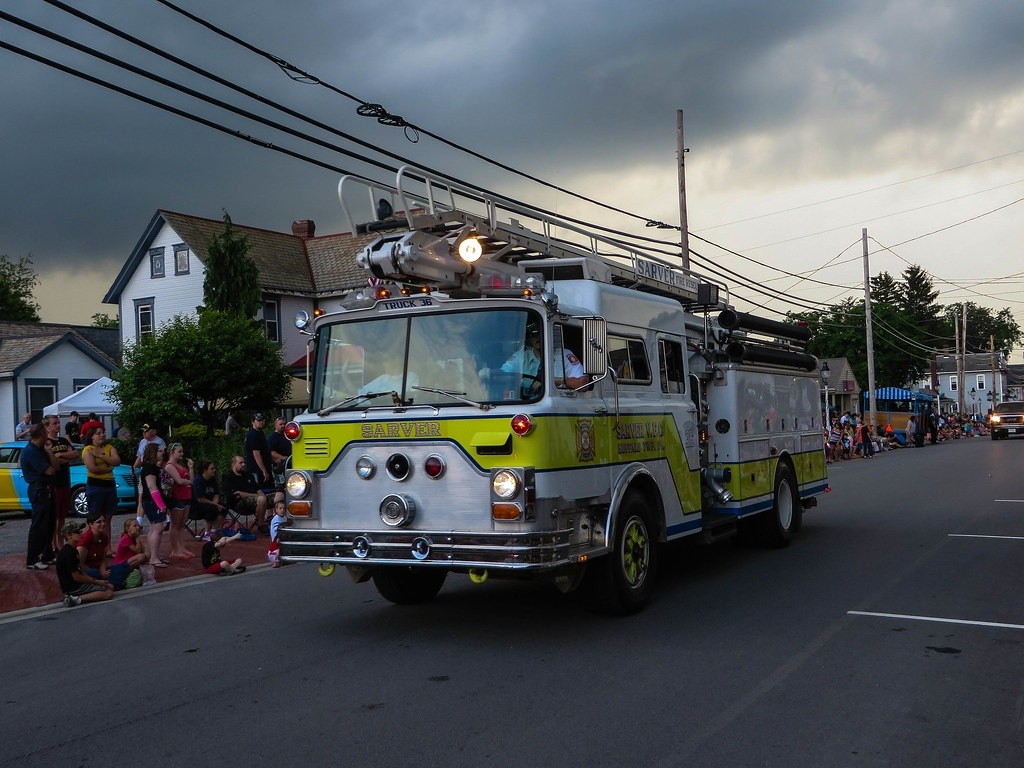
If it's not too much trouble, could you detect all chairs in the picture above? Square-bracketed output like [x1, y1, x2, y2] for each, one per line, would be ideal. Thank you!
[890, 402, 898, 412]
[220, 507, 260, 532]
[183, 512, 208, 542]
[899, 402, 908, 412]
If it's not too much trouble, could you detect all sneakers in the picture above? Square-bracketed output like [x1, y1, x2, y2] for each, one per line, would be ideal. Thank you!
[41, 557, 57, 564]
[27, 561, 48, 570]
[64, 593, 77, 607]
[203, 532, 212, 541]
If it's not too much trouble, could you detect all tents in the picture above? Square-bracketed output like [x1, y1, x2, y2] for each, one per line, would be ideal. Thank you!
[43, 377, 123, 438]
[863, 386, 933, 413]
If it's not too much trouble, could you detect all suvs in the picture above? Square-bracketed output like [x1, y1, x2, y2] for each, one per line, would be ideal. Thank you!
[986, 401, 1024, 439]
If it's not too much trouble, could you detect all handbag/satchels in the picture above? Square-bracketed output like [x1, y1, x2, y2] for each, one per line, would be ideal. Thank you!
[158, 463, 178, 499]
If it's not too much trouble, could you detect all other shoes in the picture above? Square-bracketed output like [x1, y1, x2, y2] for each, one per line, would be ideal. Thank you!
[235, 566, 246, 573]
[264, 515, 274, 525]
[257, 524, 269, 536]
[170, 551, 196, 560]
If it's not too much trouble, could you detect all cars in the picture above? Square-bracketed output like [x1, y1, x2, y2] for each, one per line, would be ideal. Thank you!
[0, 441, 142, 518]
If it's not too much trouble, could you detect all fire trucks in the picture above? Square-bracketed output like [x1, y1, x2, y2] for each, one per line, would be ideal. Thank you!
[272, 164, 842, 617]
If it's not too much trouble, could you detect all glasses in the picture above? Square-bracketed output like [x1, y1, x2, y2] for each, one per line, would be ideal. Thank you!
[70, 530, 80, 534]
[94, 519, 106, 524]
[531, 331, 544, 337]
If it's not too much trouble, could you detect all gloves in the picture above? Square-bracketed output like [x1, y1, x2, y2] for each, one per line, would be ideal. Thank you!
[479, 367, 490, 380]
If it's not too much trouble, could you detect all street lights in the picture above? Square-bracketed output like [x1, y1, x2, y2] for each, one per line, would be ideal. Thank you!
[820, 360, 831, 430]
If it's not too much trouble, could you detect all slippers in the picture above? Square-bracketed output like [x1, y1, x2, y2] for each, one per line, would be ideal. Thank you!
[147, 561, 168, 568]
[148, 558, 170, 565]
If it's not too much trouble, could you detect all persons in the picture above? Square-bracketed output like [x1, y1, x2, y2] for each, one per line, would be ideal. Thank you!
[41, 415, 80, 553]
[822, 410, 905, 462]
[225, 410, 240, 435]
[56, 512, 147, 607]
[270, 499, 287, 540]
[79, 413, 105, 444]
[80, 421, 121, 554]
[479, 324, 588, 399]
[342, 348, 419, 405]
[16, 412, 32, 441]
[187, 461, 227, 538]
[132, 413, 170, 533]
[267, 416, 292, 471]
[201, 530, 246, 576]
[64, 410, 82, 444]
[905, 415, 917, 447]
[243, 413, 276, 522]
[221, 455, 284, 535]
[140, 443, 170, 569]
[20, 423, 59, 570]
[929, 409, 991, 444]
[164, 443, 196, 560]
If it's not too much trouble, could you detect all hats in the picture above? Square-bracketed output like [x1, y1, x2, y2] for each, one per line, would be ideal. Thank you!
[254, 413, 265, 420]
[70, 411, 79, 416]
[61, 521, 86, 535]
[140, 423, 156, 432]
